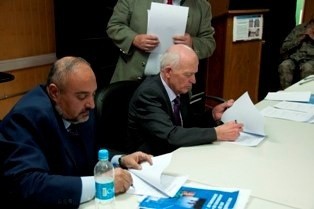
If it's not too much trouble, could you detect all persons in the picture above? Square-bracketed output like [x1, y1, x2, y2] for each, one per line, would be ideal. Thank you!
[106, 0, 216, 84]
[0, 56, 154, 209]
[129, 44, 244, 157]
[278, 19, 314, 90]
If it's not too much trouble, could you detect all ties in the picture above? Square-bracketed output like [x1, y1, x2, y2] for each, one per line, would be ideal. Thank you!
[167, 0, 173, 5]
[173, 96, 181, 126]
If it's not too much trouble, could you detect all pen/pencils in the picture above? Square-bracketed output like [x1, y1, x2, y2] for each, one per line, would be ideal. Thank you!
[113, 162, 135, 188]
[235, 119, 237, 124]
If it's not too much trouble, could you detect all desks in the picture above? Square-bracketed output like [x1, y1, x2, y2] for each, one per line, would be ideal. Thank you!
[79, 74, 314, 209]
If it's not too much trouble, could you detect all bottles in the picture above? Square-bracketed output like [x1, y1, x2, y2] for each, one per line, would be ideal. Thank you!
[95, 149, 116, 209]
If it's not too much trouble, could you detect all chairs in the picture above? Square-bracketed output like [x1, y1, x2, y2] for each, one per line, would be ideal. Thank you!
[179, 58, 226, 128]
[95, 79, 143, 154]
